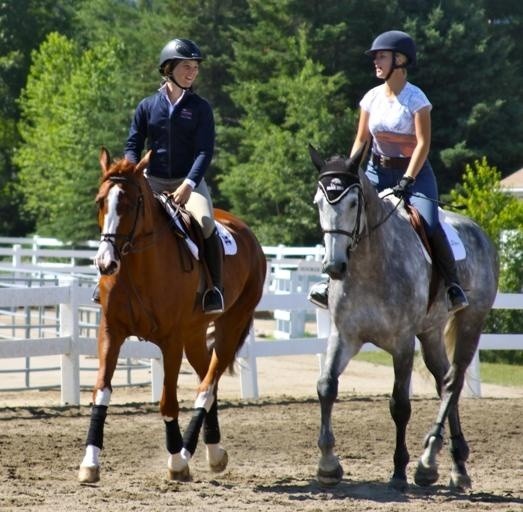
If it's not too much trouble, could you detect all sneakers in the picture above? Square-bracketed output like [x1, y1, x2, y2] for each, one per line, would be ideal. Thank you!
[393, 176, 415, 199]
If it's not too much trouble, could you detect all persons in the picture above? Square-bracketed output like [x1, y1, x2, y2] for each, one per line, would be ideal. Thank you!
[90, 35, 225, 311]
[305, 29, 466, 309]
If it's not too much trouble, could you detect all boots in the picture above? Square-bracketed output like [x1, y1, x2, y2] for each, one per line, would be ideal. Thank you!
[311, 283, 329, 304]
[94, 291, 100, 301]
[427, 221, 465, 306]
[204, 227, 224, 310]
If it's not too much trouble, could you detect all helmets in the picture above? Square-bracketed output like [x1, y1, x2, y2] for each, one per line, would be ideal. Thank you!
[364, 30, 416, 68]
[159, 39, 203, 69]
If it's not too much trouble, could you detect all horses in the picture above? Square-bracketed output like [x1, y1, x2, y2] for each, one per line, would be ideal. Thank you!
[306, 140, 501, 493]
[77, 145, 267, 485]
